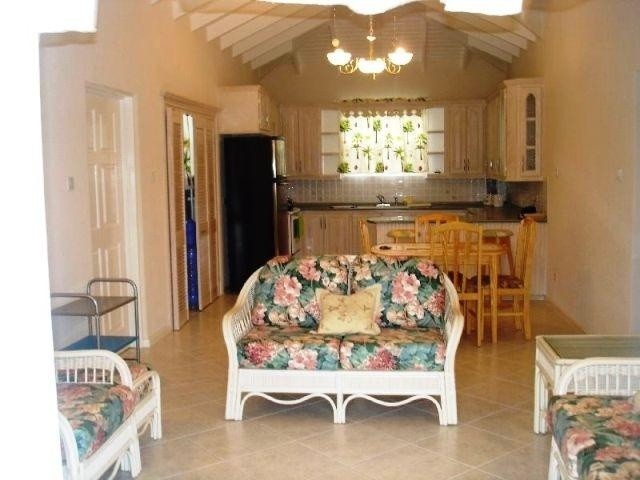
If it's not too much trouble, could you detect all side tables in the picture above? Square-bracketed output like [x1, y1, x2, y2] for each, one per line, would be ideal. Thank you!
[532, 335, 640, 435]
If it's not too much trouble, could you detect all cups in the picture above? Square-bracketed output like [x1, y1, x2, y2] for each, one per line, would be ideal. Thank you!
[494, 194, 503, 207]
[483, 193, 494, 206]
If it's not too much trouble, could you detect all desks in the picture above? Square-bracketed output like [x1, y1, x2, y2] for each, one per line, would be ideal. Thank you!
[371, 240, 505, 345]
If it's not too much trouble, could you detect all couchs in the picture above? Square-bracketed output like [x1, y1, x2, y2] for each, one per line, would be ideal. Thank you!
[221, 253, 464, 427]
[547, 355, 640, 480]
[55, 349, 160, 478]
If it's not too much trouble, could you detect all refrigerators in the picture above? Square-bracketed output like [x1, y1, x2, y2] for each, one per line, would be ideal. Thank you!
[220, 134, 288, 295]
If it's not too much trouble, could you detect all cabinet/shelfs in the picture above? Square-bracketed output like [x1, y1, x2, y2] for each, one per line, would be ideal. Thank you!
[425, 101, 446, 180]
[352, 214, 377, 256]
[51, 277, 141, 366]
[485, 87, 543, 183]
[446, 104, 485, 179]
[303, 213, 350, 257]
[257, 87, 283, 137]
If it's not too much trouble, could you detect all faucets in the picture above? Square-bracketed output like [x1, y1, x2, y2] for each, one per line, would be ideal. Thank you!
[375, 194, 390, 207]
[393, 192, 399, 206]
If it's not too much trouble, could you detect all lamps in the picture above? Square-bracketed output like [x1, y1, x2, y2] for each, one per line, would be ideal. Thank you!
[326, 15, 414, 81]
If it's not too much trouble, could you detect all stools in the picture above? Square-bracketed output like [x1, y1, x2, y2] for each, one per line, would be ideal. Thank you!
[387, 229, 422, 243]
[481, 228, 521, 331]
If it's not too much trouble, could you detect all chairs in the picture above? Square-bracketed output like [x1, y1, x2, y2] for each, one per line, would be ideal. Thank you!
[467, 217, 536, 341]
[415, 213, 459, 243]
[430, 221, 482, 348]
